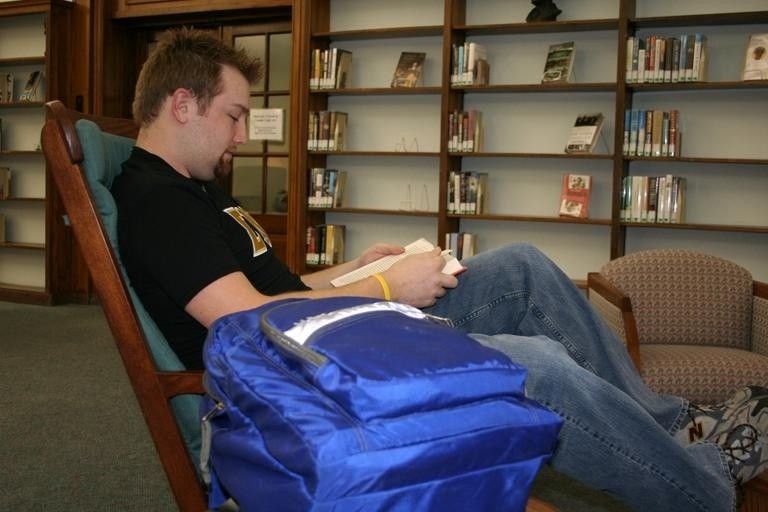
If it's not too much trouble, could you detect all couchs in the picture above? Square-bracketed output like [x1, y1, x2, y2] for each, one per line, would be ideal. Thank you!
[585, 249, 768, 403]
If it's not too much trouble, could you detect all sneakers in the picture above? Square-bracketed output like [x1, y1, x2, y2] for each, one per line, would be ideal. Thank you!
[671, 386, 768, 447]
[708, 396, 768, 485]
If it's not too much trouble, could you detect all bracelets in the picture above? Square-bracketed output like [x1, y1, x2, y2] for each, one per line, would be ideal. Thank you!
[373, 272, 391, 300]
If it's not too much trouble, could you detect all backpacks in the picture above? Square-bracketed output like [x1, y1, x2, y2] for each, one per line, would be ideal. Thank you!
[202, 297, 563, 511]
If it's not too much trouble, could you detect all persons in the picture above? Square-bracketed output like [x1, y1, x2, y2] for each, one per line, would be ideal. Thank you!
[111, 29, 767, 511]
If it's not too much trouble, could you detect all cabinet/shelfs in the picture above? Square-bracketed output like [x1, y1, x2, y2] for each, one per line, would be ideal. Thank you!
[305, 22, 452, 283]
[612, 1, 768, 296]
[434, 0, 635, 286]
[0, 52, 48, 307]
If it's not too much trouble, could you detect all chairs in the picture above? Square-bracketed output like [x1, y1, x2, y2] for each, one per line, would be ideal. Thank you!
[38, 91, 565, 512]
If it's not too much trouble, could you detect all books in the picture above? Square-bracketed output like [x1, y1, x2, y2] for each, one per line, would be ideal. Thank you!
[447, 232, 475, 265]
[447, 170, 487, 214]
[626, 33, 706, 82]
[560, 174, 592, 218]
[541, 41, 576, 82]
[307, 111, 347, 151]
[390, 52, 426, 88]
[310, 48, 351, 89]
[450, 42, 489, 86]
[622, 109, 681, 157]
[328, 238, 467, 288]
[309, 168, 345, 209]
[742, 34, 767, 81]
[619, 174, 686, 222]
[0, 73, 13, 102]
[0, 214, 7, 244]
[448, 108, 482, 152]
[305, 225, 344, 266]
[564, 112, 606, 152]
[0, 167, 11, 197]
[18, 72, 45, 101]
